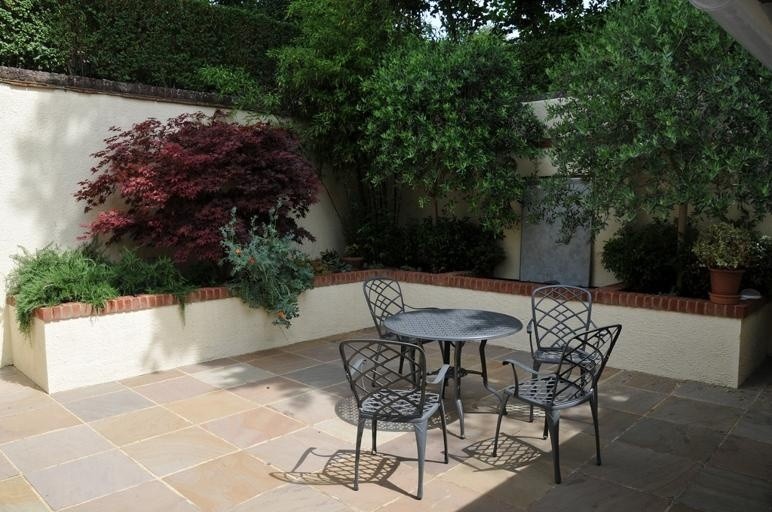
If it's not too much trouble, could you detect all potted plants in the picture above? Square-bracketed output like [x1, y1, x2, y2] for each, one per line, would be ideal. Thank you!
[690, 224, 767, 306]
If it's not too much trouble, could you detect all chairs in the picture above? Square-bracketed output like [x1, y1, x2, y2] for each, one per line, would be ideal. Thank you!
[492, 324, 622, 485]
[362, 276, 441, 398]
[526, 285, 605, 423]
[338, 339, 449, 501]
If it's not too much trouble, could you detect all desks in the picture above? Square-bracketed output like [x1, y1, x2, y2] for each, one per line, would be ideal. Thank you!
[383, 308, 523, 439]
